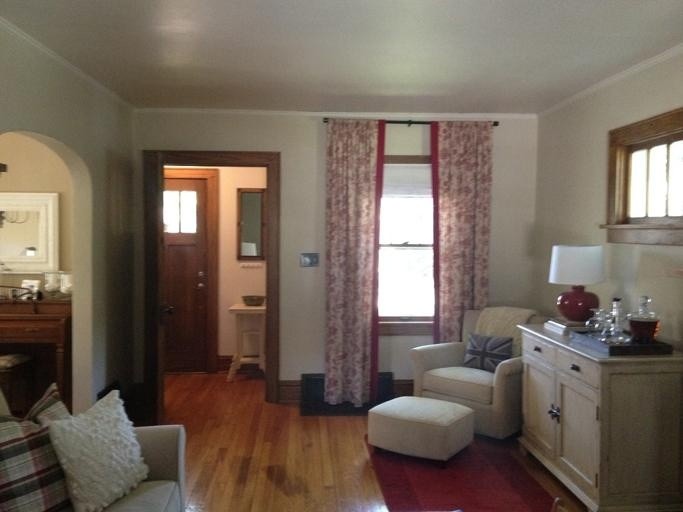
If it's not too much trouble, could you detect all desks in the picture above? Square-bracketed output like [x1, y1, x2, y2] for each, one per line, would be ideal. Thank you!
[0, 291, 72, 409]
[226, 303, 267, 383]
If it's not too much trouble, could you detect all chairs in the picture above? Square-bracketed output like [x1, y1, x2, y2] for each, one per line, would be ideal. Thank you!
[0, 391, 186, 512]
[411, 307, 549, 444]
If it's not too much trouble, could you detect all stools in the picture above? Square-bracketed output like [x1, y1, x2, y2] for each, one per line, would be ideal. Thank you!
[0, 349, 33, 412]
[365, 395, 476, 463]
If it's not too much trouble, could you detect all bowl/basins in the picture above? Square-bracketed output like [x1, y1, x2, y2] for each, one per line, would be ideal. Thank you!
[241, 296, 265, 305]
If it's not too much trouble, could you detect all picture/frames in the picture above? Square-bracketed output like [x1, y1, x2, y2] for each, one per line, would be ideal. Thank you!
[20, 279, 41, 300]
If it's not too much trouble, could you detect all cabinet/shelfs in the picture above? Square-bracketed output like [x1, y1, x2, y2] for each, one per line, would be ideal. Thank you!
[515, 323, 683, 512]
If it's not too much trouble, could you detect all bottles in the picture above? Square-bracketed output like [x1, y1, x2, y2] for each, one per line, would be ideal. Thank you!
[585, 294, 652, 343]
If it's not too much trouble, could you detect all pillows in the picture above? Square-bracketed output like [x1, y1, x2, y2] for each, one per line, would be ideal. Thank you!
[0, 384, 73, 510]
[48, 388, 150, 511]
[465, 332, 514, 373]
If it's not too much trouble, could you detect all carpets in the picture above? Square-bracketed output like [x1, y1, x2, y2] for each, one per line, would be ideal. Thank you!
[362, 430, 562, 512]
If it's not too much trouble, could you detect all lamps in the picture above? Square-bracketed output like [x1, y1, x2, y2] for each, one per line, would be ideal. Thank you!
[0, 211, 35, 228]
[543, 243, 608, 337]
[24, 246, 37, 256]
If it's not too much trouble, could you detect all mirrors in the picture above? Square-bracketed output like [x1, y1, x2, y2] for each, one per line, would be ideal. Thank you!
[0, 190, 60, 274]
[235, 186, 267, 261]
